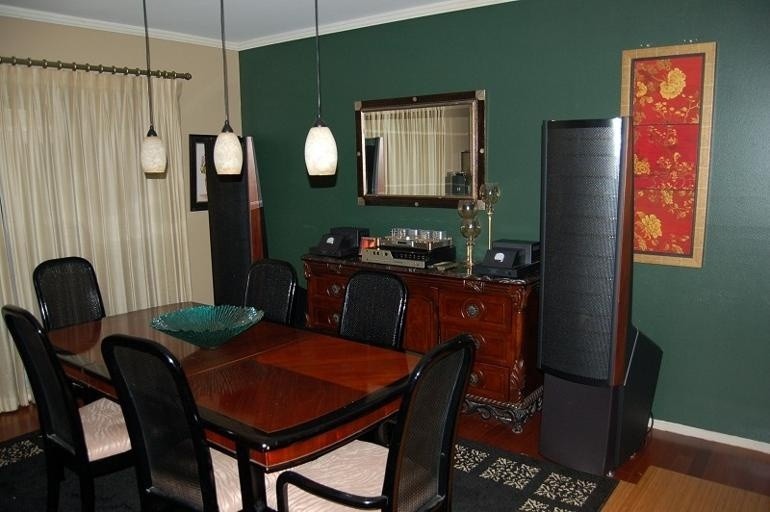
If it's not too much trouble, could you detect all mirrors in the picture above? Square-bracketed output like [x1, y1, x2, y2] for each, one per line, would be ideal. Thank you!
[350, 89, 488, 210]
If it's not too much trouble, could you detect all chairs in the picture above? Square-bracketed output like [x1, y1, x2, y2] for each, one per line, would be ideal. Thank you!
[2, 302, 139, 512]
[259, 332, 477, 512]
[29, 256, 106, 409]
[240, 256, 299, 329]
[338, 266, 409, 353]
[98, 329, 249, 512]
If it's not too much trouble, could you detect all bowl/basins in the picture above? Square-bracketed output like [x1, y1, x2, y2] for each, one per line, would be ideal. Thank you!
[146, 304, 265, 351]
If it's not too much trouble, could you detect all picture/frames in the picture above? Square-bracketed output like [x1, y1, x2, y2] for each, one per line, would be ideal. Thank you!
[186, 132, 211, 213]
[615, 39, 718, 270]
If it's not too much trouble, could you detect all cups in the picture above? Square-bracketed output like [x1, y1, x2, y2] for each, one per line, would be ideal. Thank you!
[377, 227, 448, 249]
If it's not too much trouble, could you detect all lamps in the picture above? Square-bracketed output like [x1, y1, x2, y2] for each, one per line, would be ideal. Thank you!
[137, 0, 170, 177]
[211, 0, 245, 174]
[302, 0, 340, 177]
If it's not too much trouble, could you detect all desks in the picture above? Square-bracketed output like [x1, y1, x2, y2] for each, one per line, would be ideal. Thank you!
[37, 298, 430, 512]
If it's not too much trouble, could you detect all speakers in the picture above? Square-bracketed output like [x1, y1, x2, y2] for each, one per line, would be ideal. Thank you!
[538, 117, 663, 478]
[205, 136, 268, 309]
[364, 137, 385, 195]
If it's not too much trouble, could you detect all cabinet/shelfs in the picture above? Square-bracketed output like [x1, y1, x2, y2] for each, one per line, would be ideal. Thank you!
[298, 245, 546, 438]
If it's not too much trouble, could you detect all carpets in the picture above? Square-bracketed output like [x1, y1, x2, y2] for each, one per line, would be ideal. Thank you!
[0, 415, 622, 512]
